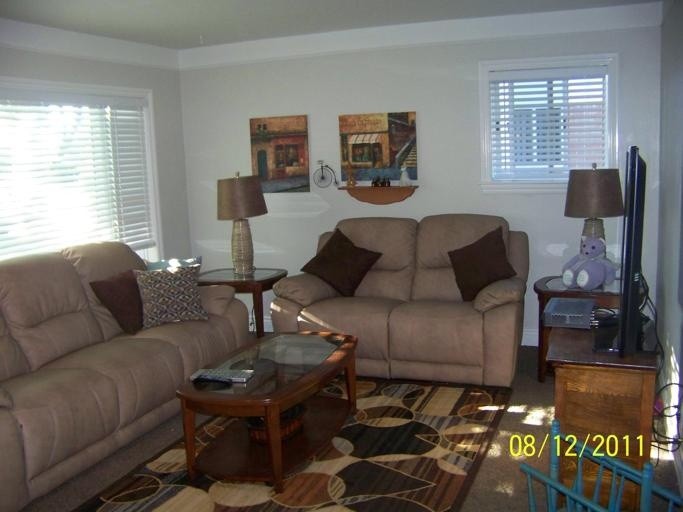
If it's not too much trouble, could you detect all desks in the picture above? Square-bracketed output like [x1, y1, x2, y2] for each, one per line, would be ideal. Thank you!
[197, 269, 288, 338]
[533, 276, 624, 382]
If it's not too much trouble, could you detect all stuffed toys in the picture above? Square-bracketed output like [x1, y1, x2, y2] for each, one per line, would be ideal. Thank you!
[561, 235, 615, 291]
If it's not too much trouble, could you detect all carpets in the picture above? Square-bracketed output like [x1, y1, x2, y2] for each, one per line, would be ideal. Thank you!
[72, 382, 513, 512]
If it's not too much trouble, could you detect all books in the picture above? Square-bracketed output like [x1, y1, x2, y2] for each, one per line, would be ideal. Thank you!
[189, 368, 255, 384]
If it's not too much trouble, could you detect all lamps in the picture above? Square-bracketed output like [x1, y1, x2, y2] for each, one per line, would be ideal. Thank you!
[564, 163, 626, 287]
[217, 171, 268, 275]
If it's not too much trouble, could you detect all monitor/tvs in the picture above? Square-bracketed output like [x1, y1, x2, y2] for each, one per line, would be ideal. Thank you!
[597, 146, 658, 354]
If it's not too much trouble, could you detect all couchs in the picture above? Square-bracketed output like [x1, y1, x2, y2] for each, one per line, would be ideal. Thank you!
[270, 214, 529, 388]
[0, 242, 249, 512]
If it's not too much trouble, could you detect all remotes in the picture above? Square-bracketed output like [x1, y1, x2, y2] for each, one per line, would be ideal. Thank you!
[192, 375, 232, 386]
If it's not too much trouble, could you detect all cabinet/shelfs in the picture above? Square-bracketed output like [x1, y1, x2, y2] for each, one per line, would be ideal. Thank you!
[546, 329, 660, 511]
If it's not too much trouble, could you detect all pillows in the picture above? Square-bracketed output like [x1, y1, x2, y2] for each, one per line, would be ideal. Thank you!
[91, 270, 144, 334]
[146, 256, 202, 269]
[448, 226, 516, 302]
[135, 263, 209, 327]
[300, 229, 381, 297]
